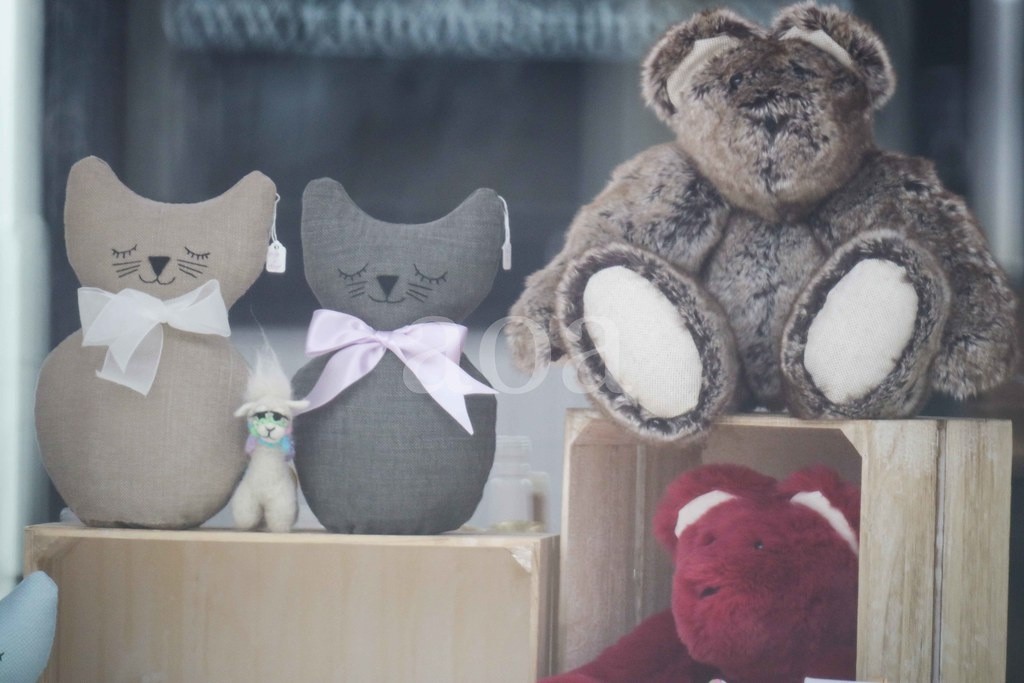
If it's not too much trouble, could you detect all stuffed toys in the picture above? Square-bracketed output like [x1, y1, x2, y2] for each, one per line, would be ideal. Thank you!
[506, 0, 1024, 451]
[231, 357, 309, 532]
[34, 156, 278, 531]
[289, 178, 504, 536]
[542, 464, 861, 683]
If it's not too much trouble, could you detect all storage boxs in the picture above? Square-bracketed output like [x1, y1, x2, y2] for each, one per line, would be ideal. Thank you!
[558, 408, 1013, 683]
